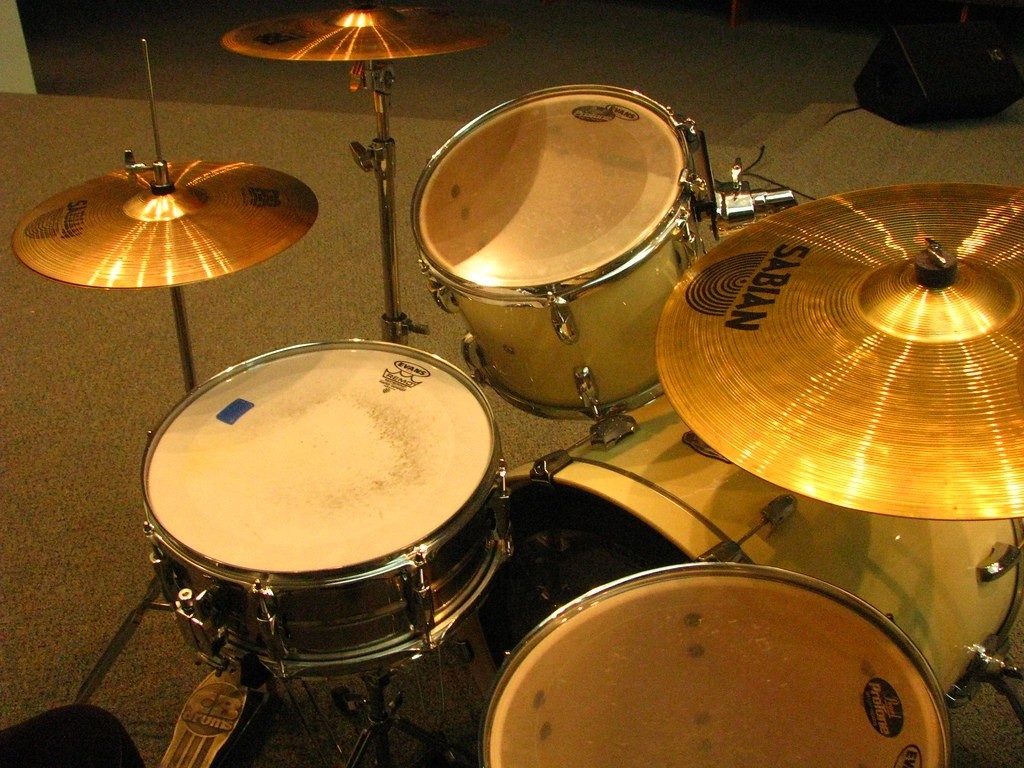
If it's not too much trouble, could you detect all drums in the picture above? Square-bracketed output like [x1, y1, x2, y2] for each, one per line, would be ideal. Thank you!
[410, 82, 712, 422]
[653, 181, 1024, 524]
[476, 391, 1023, 712]
[475, 559, 955, 768]
[137, 338, 513, 679]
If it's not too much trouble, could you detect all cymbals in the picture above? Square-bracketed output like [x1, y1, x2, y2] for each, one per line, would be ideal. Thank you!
[218, 5, 513, 62]
[11, 161, 319, 290]
